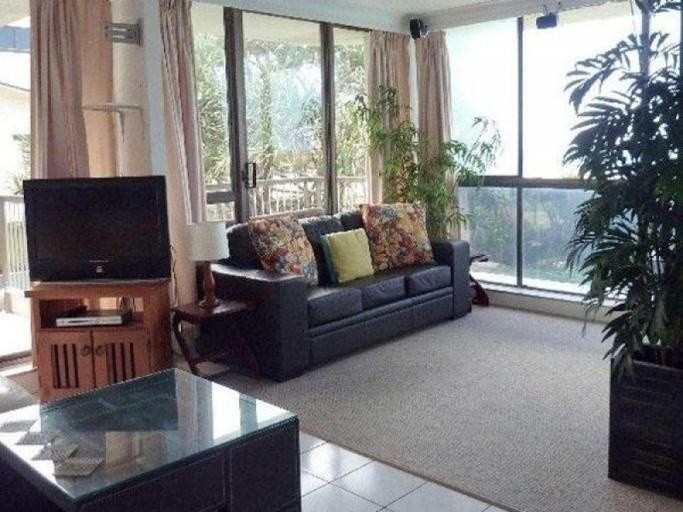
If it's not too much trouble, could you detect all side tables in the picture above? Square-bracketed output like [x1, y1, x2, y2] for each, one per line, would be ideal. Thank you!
[468, 255, 491, 307]
[171, 300, 262, 383]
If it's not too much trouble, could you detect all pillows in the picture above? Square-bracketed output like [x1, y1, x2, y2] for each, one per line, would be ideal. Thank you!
[325, 228, 374, 283]
[318, 236, 336, 283]
[248, 213, 318, 287]
[359, 201, 435, 269]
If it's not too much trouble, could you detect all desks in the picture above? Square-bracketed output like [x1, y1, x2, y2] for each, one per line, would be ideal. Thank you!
[0, 368, 300, 511]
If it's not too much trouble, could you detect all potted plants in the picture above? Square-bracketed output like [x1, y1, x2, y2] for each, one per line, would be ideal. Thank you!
[563, 0, 683, 500]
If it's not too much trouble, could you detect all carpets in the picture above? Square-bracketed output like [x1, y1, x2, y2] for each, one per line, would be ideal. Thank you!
[7, 304, 683, 512]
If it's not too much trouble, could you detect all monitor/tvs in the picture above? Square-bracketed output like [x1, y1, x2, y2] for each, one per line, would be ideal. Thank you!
[21, 173, 173, 289]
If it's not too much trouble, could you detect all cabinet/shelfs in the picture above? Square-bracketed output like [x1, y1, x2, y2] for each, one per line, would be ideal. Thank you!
[24, 278, 171, 402]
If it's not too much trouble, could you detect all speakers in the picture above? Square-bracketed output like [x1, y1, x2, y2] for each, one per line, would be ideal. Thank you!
[409, 17, 422, 40]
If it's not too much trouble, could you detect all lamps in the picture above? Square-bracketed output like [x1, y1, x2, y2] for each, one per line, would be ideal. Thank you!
[535, 2, 561, 28]
[190, 220, 230, 309]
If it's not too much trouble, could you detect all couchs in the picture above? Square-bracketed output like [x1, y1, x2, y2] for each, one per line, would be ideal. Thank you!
[196, 210, 471, 380]
